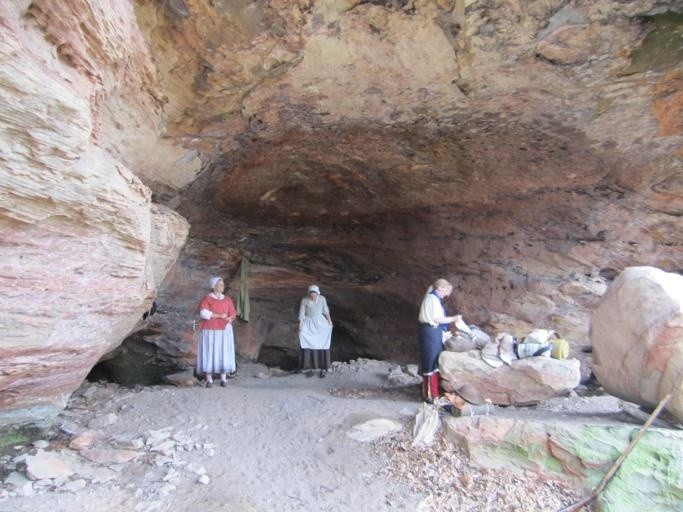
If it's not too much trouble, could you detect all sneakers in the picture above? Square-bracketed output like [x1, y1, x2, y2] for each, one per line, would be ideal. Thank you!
[319, 371, 326, 378]
[220, 381, 227, 386]
[205, 382, 214, 387]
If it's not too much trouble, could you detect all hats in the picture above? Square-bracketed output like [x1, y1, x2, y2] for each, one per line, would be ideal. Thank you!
[307, 284, 321, 295]
[452, 381, 484, 406]
[209, 277, 222, 290]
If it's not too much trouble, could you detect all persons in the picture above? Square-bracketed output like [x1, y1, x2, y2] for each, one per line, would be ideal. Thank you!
[297, 283, 333, 378]
[194, 276, 237, 388]
[417, 277, 462, 405]
